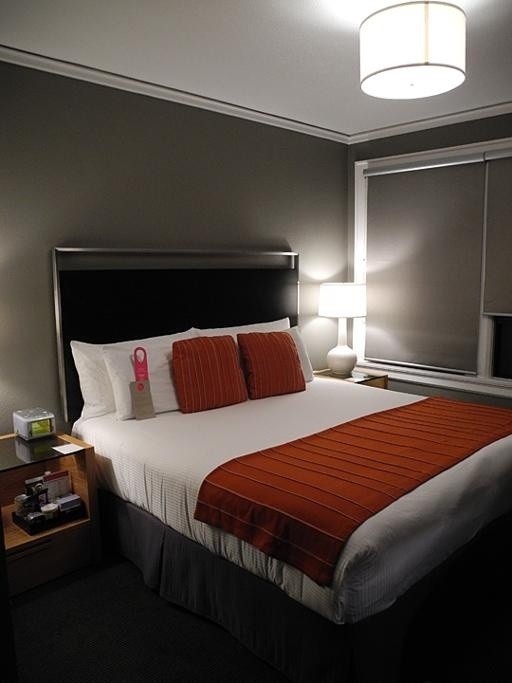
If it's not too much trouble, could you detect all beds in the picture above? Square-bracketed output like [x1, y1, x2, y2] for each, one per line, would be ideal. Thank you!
[54, 247, 512, 683]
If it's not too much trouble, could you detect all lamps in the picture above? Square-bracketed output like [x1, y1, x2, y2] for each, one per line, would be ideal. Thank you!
[359, 0, 468, 101]
[318, 282, 367, 374]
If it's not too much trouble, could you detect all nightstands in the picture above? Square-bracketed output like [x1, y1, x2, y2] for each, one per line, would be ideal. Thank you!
[317, 367, 389, 389]
[0, 428, 99, 597]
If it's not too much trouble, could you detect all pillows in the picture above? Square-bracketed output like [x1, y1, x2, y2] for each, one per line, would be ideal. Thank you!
[69, 317, 313, 417]
